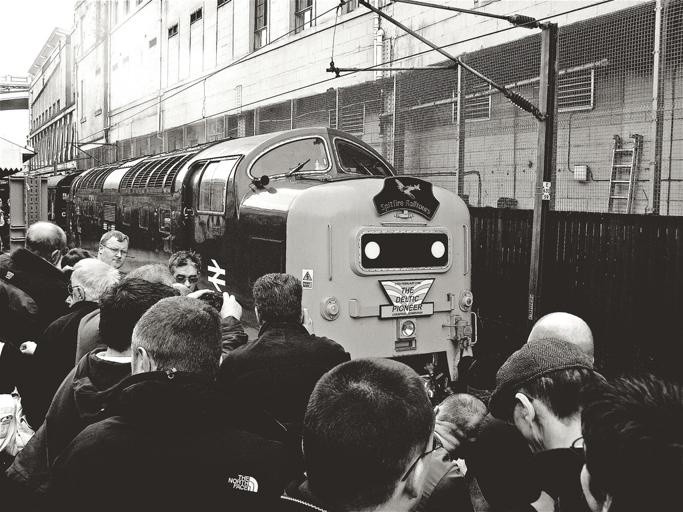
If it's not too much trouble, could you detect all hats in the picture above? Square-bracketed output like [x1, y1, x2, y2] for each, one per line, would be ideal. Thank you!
[483, 339, 596, 418]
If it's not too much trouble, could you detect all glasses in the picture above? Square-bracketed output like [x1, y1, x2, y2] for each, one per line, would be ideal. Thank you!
[103, 244, 128, 255]
[66, 284, 81, 294]
[176, 274, 201, 283]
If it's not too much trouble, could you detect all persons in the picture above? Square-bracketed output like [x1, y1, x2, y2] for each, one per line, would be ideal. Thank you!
[1, 192, 680, 510]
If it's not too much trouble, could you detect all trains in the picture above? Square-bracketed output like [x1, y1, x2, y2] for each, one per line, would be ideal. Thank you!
[46, 125, 479, 401]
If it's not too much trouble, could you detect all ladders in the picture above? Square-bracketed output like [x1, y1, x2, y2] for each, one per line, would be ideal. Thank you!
[608, 134, 643, 214]
